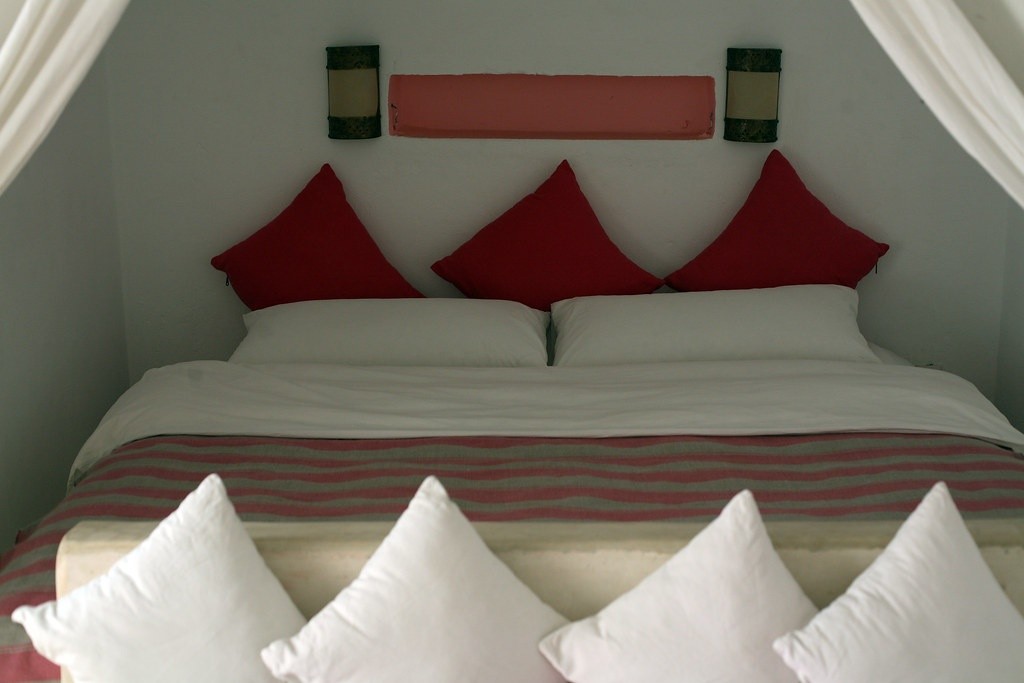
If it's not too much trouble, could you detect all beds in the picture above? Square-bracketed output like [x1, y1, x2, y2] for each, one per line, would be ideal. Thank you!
[0, 138, 1024, 683]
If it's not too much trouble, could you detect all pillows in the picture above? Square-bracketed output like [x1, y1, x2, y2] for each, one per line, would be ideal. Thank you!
[10, 473, 308, 683]
[227, 298, 552, 368]
[209, 163, 426, 311]
[772, 481, 1024, 683]
[553, 284, 880, 372]
[430, 159, 662, 313]
[260, 474, 573, 683]
[538, 489, 820, 683]
[663, 148, 889, 291]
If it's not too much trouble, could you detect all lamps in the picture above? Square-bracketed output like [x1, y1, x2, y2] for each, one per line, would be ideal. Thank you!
[723, 48, 783, 142]
[325, 46, 384, 140]
